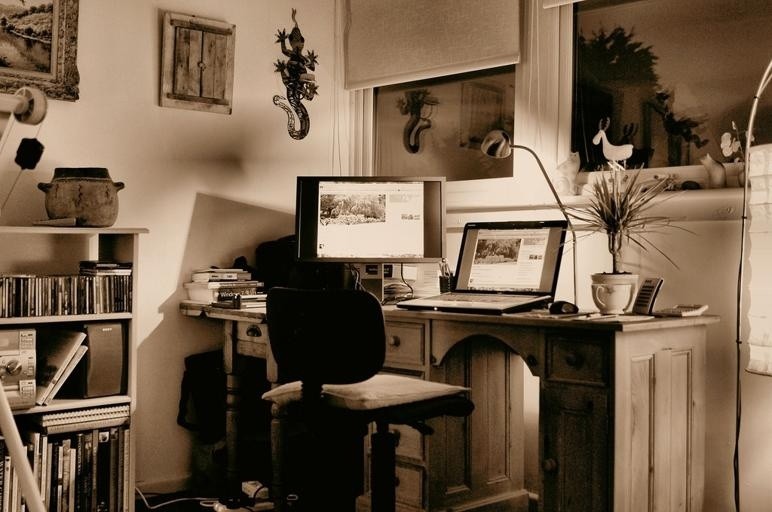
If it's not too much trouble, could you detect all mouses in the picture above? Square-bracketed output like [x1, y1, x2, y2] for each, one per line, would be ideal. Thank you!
[549, 301, 578, 316]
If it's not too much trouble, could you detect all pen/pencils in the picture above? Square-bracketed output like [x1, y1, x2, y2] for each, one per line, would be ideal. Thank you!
[438, 258, 454, 277]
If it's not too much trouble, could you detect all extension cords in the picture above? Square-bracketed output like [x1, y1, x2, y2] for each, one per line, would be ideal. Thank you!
[212, 494, 274, 511]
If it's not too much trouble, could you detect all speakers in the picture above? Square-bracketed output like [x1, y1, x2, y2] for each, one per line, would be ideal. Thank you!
[70, 323, 123, 397]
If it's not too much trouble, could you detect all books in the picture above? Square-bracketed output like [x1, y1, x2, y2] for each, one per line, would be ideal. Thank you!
[184, 267, 269, 310]
[0, 405, 129, 512]
[35, 332, 89, 405]
[0, 261, 132, 319]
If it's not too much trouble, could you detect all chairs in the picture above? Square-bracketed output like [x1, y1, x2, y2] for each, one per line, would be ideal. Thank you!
[261, 287, 475, 512]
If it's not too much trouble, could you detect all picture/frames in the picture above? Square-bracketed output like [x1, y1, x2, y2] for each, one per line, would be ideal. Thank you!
[1, 0, 80, 101]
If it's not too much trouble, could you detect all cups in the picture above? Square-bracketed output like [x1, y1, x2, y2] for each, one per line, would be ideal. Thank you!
[592, 284, 633, 316]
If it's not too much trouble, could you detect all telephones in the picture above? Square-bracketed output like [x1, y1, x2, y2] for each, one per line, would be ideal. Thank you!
[384, 282, 412, 292]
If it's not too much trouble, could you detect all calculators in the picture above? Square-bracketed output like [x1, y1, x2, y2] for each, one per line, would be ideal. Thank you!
[653, 304, 709, 316]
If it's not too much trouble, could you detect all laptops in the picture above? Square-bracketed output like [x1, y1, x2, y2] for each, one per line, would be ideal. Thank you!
[395, 220, 569, 315]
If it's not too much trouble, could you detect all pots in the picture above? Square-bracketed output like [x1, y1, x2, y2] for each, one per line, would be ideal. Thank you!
[38, 168, 124, 227]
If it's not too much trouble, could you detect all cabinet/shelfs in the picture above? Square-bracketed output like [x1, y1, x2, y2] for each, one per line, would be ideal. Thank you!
[0, 226, 149, 512]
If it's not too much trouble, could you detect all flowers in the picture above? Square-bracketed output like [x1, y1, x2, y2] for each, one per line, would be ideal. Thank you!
[558, 164, 702, 274]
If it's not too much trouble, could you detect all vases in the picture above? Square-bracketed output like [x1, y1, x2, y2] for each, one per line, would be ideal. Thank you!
[590, 274, 639, 314]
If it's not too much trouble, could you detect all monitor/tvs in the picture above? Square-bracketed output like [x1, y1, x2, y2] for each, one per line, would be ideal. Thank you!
[294, 176, 448, 302]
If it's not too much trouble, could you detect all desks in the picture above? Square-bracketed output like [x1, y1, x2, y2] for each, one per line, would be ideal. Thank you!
[178, 301, 722, 511]
